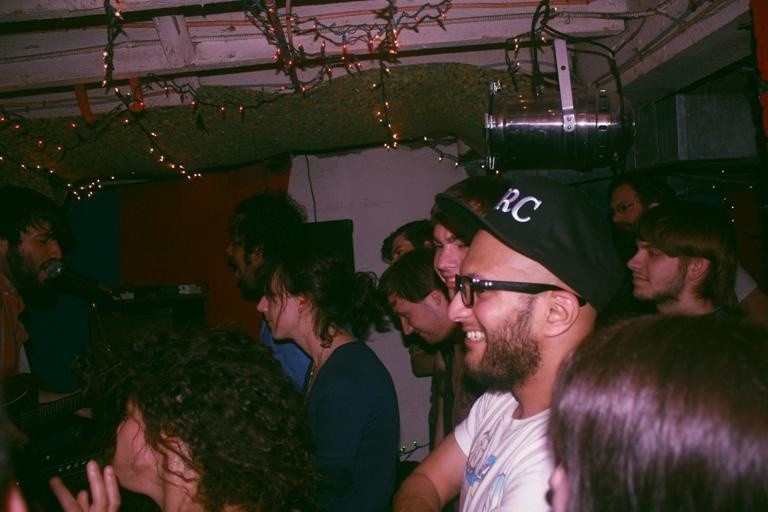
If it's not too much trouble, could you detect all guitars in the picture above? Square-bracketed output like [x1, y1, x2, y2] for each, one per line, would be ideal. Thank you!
[0, 369, 109, 478]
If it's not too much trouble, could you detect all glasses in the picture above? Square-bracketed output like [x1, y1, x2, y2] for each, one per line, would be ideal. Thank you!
[455, 274, 587, 308]
[616, 201, 642, 212]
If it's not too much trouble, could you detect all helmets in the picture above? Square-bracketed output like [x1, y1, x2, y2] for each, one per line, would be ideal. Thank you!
[435, 177, 506, 218]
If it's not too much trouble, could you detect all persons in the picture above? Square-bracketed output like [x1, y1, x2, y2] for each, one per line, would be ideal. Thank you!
[0, 185, 401, 511]
[375, 169, 767, 512]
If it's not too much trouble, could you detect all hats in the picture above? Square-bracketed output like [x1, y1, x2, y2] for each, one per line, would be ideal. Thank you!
[474, 177, 627, 312]
